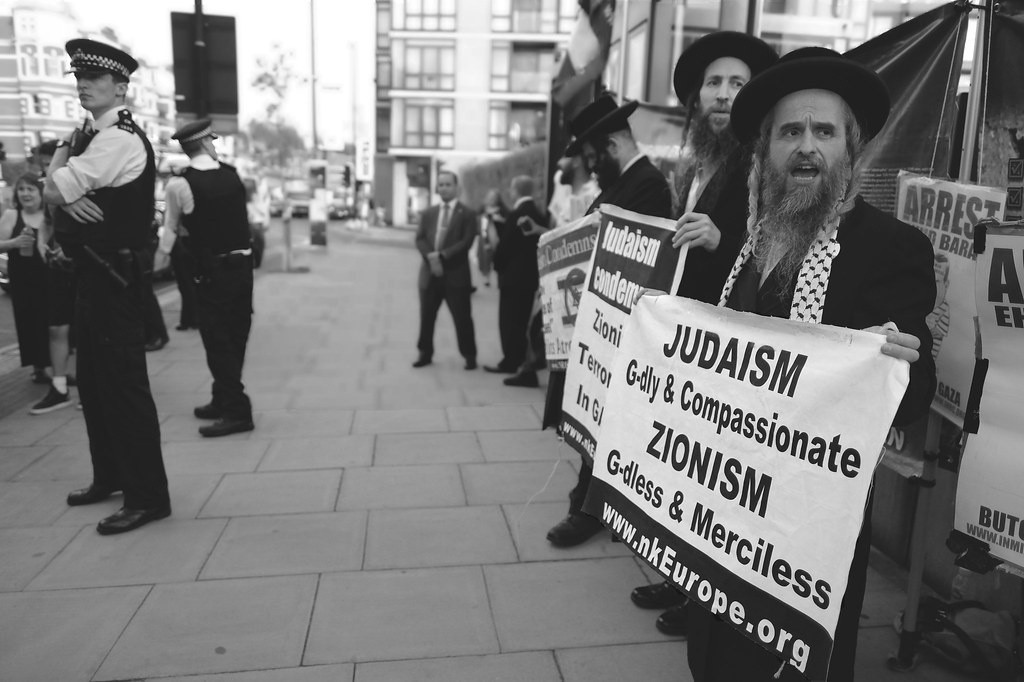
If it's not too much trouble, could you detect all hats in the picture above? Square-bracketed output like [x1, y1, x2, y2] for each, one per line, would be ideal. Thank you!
[727, 48, 891, 146]
[559, 95, 642, 158]
[171, 118, 219, 143]
[673, 30, 781, 113]
[64, 38, 139, 83]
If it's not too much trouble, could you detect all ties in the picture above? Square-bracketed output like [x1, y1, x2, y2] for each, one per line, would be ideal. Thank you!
[436, 206, 450, 251]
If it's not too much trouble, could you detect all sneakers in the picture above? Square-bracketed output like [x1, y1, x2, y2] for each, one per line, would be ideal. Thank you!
[29, 383, 73, 415]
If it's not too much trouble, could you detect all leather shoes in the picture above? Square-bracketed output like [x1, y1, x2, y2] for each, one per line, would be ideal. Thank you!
[195, 399, 227, 418]
[66, 479, 124, 506]
[655, 608, 690, 637]
[199, 414, 255, 437]
[96, 504, 172, 535]
[547, 512, 603, 546]
[630, 580, 687, 611]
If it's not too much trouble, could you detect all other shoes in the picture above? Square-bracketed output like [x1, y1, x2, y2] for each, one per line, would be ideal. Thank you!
[465, 358, 477, 370]
[412, 355, 432, 366]
[484, 363, 517, 374]
[503, 373, 539, 387]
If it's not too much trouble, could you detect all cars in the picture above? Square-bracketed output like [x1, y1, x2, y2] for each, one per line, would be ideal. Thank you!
[0, 147, 358, 297]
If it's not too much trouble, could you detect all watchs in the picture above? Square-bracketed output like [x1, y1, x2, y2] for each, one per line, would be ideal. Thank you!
[56, 140, 70, 148]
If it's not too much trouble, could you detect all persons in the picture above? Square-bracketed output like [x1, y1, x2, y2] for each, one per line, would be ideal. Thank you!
[630, 31, 778, 637]
[485, 175, 543, 388]
[546, 97, 673, 548]
[162, 119, 256, 437]
[475, 187, 506, 286]
[0, 140, 83, 413]
[411, 171, 478, 370]
[504, 142, 602, 388]
[43, 38, 172, 535]
[632, 46, 937, 682]
